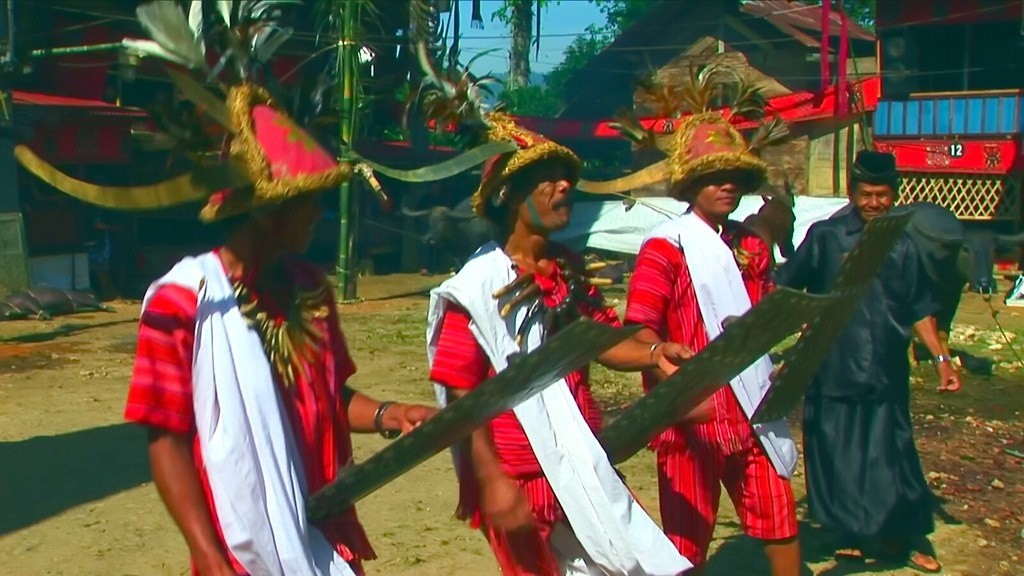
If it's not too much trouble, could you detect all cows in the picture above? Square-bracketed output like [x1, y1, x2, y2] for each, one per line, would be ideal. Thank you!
[897, 202, 1024, 369]
[0, 290, 117, 320]
[402, 195, 499, 274]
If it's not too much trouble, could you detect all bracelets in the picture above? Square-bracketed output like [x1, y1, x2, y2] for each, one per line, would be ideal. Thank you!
[651, 341, 664, 368]
[933, 354, 950, 365]
[373, 401, 403, 439]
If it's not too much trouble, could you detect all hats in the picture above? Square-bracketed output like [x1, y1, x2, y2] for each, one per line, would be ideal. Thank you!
[577, 42, 793, 204]
[851, 150, 897, 185]
[348, 41, 582, 223]
[11, 0, 357, 226]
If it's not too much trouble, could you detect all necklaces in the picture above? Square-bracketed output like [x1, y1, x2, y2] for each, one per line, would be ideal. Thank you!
[491, 251, 624, 351]
[222, 246, 332, 388]
[726, 231, 764, 272]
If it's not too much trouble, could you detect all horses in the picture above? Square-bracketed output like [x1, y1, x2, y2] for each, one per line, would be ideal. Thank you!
[742, 194, 796, 272]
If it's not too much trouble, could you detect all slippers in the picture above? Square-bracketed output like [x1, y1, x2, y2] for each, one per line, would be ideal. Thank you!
[833, 545, 865, 566]
[903, 549, 942, 573]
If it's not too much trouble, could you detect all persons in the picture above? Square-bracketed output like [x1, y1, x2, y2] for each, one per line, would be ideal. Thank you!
[130, 119, 449, 576]
[426, 136, 697, 576]
[623, 121, 813, 576]
[769, 149, 964, 572]
